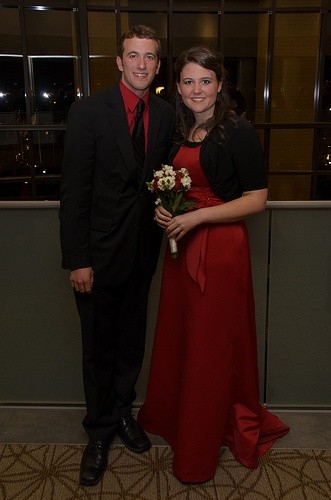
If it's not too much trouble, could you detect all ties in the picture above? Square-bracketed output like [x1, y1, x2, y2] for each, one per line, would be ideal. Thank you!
[131, 99, 146, 183]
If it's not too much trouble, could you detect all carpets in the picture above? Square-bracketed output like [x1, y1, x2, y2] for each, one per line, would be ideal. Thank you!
[0, 443, 331, 500]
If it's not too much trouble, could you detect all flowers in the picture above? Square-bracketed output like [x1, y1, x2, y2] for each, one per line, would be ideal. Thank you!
[146, 165, 196, 259]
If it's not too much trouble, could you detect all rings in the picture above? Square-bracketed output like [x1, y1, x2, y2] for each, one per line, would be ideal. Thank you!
[153, 214, 158, 221]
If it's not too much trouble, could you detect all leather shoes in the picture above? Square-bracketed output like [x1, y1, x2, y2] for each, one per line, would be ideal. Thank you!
[117, 416, 152, 452]
[79, 438, 110, 487]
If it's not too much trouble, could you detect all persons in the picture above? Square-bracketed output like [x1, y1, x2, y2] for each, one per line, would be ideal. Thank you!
[58, 24, 176, 487]
[153, 45, 291, 485]
